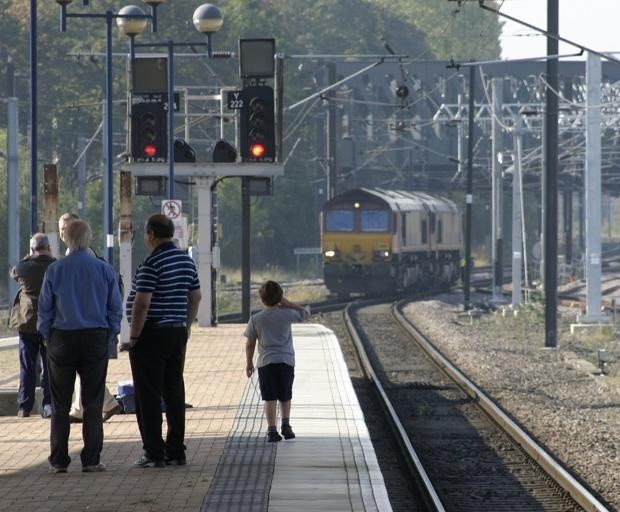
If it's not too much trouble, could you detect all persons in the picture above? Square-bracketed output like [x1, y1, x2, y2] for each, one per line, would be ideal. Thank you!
[119, 214, 201, 467]
[244, 281, 311, 442]
[10, 233, 57, 418]
[36, 220, 123, 472]
[59, 213, 123, 423]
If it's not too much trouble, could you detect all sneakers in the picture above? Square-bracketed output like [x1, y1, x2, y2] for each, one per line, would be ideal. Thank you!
[48, 464, 67, 472]
[267, 431, 282, 442]
[82, 462, 106, 473]
[134, 447, 187, 467]
[17, 407, 30, 417]
[281, 425, 296, 440]
[40, 404, 51, 418]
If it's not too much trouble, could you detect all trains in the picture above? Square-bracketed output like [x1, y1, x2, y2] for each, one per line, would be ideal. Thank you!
[320, 186, 465, 302]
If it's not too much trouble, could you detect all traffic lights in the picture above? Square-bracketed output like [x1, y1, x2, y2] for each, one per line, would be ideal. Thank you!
[237, 84, 274, 159]
[129, 99, 168, 160]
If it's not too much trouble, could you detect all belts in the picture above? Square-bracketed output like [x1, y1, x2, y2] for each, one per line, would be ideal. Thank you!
[148, 322, 188, 330]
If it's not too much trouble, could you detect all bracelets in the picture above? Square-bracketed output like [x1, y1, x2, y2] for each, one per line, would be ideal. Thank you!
[130, 337, 138, 340]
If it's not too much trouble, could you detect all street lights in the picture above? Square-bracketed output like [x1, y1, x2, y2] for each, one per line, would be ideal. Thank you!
[116, 3, 225, 203]
